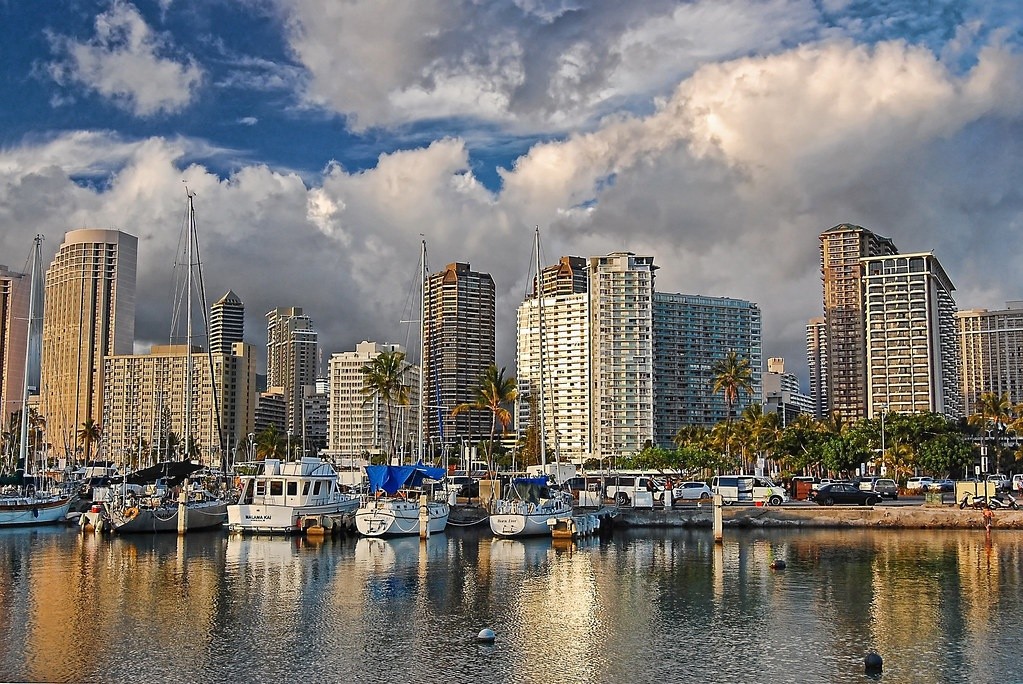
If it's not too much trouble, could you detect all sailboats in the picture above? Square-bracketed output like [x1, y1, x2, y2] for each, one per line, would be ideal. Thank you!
[355, 233, 450, 537]
[488, 225, 574, 536]
[0, 181, 235, 536]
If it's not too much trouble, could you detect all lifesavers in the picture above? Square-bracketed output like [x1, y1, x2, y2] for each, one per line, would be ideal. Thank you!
[125, 507, 138, 519]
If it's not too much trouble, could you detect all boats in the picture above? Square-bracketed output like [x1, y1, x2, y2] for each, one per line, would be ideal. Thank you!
[222, 456, 359, 533]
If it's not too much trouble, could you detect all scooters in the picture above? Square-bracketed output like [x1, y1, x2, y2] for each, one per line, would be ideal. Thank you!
[959, 490, 986, 509]
[988, 491, 1020, 511]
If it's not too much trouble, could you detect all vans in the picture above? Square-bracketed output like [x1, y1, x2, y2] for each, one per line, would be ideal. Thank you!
[711, 475, 789, 506]
[859, 477, 881, 492]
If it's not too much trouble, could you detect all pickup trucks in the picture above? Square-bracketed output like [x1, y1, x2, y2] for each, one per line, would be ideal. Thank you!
[604, 476, 683, 506]
[987, 474, 1023, 491]
[792, 476, 860, 490]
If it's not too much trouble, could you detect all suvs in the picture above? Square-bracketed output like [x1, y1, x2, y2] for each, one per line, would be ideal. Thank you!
[873, 479, 898, 500]
[907, 477, 954, 491]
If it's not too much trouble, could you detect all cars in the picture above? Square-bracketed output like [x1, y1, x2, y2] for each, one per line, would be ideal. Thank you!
[810, 483, 883, 506]
[675, 481, 712, 500]
[440, 476, 474, 492]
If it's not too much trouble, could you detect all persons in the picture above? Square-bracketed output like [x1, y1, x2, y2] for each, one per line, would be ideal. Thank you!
[647, 475, 657, 501]
[1017, 479, 1023, 498]
